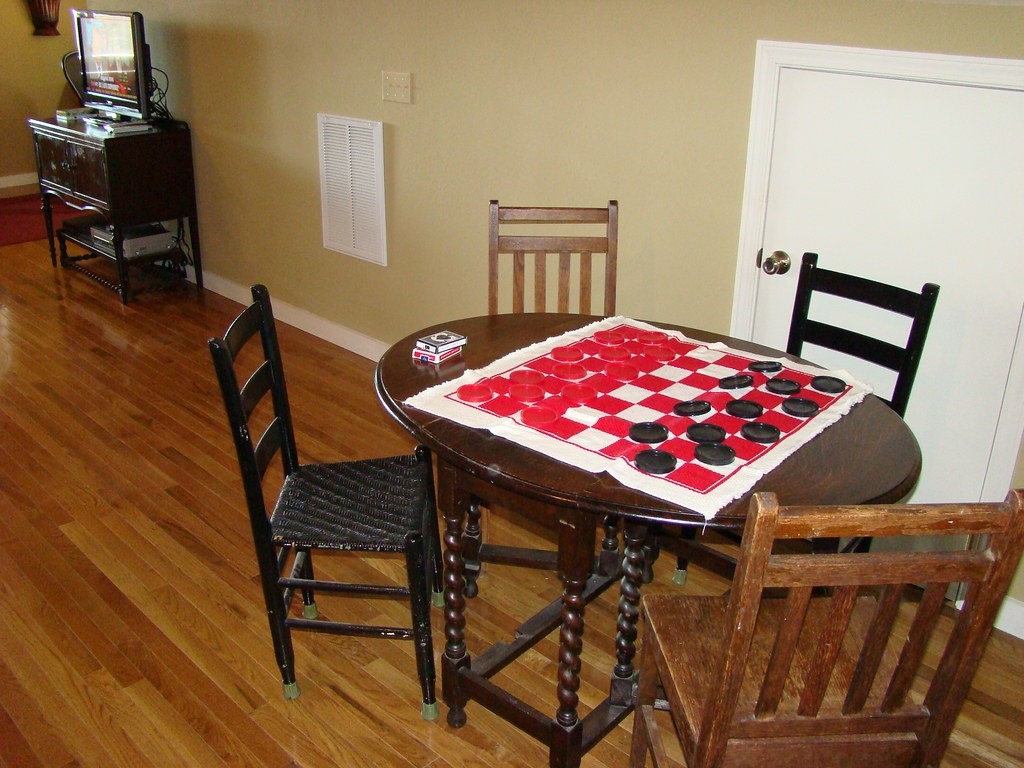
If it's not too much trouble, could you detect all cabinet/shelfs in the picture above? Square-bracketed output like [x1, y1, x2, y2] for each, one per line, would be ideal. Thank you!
[26, 113, 203, 305]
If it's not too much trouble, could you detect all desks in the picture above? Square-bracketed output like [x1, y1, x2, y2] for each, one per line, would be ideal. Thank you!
[372, 314, 922, 768]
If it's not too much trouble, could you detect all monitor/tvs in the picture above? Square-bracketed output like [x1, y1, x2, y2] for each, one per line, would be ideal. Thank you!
[73, 9, 153, 124]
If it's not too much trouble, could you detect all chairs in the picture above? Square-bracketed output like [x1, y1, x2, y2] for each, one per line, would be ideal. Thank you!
[673, 251, 940, 596]
[626, 489, 1023, 768]
[486, 197, 619, 319]
[211, 283, 442, 723]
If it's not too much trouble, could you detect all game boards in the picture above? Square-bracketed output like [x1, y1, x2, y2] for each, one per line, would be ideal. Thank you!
[401, 313, 876, 521]
